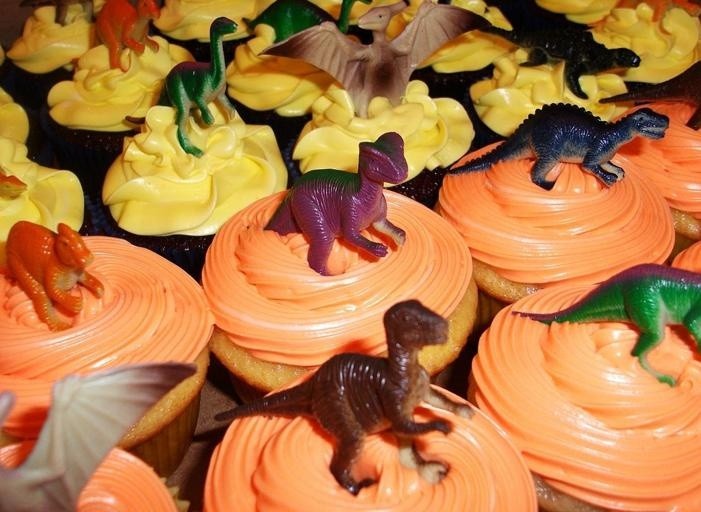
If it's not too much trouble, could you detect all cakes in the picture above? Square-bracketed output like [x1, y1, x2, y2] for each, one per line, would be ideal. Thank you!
[0, 0, 701, 512]
